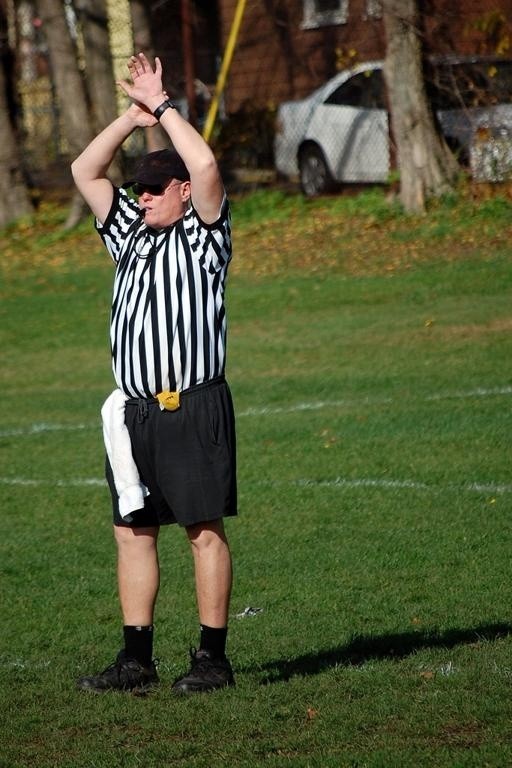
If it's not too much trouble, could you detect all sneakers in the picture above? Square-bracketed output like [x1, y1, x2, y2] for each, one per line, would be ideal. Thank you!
[170, 658, 236, 696]
[77, 658, 161, 696]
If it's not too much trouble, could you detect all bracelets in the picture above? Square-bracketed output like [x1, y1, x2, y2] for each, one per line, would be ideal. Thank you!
[152, 99, 175, 123]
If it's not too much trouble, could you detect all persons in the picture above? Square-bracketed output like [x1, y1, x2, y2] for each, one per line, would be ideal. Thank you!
[68, 50, 238, 695]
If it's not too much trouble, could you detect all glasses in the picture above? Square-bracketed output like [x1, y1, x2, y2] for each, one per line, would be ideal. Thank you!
[132, 182, 182, 196]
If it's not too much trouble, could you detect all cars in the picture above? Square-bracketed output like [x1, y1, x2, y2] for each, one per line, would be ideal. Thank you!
[273, 58, 511, 195]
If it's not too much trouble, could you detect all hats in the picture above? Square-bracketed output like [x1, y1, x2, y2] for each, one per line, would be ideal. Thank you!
[121, 149, 190, 189]
